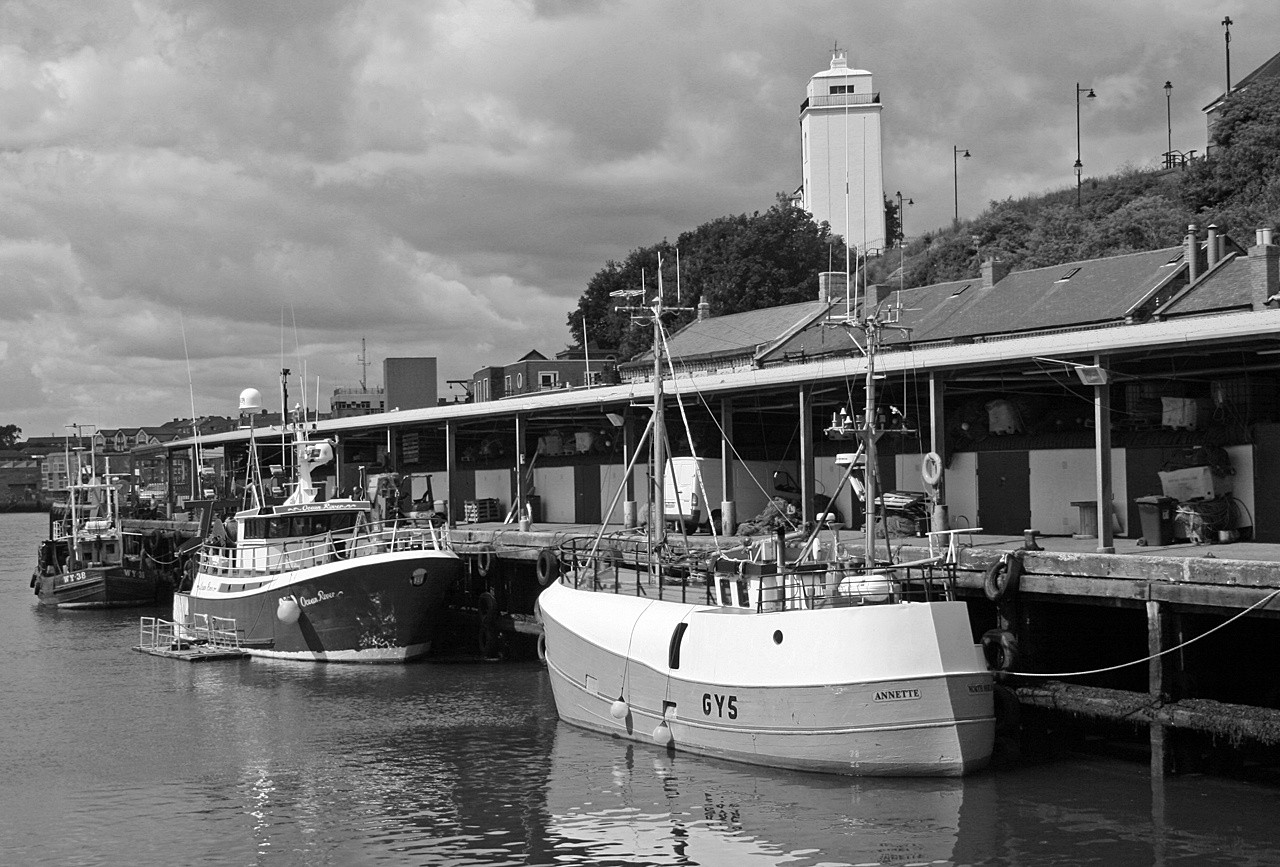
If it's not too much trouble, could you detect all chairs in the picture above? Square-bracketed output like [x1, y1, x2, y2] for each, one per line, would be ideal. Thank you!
[413, 491, 428, 502]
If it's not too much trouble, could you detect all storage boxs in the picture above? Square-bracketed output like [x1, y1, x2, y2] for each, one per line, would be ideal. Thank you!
[1156, 464, 1234, 501]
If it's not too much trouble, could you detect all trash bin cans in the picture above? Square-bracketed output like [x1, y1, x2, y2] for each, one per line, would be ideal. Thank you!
[1134, 495, 1179, 547]
[526, 495, 541, 523]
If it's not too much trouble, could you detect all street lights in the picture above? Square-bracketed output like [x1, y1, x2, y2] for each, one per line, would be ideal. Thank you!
[1073, 82, 1096, 207]
[900, 196, 914, 243]
[1163, 80, 1174, 168]
[953, 145, 971, 219]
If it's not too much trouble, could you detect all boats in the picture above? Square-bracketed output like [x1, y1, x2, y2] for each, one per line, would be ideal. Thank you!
[534, 50, 996, 778]
[173, 307, 460, 663]
[30, 424, 155, 609]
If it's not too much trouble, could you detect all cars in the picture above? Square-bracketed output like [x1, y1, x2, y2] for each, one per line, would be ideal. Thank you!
[271, 486, 283, 496]
[203, 488, 215, 499]
[128, 483, 168, 505]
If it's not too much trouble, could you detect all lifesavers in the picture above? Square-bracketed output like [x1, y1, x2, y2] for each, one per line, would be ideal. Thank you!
[921, 452, 942, 485]
[478, 544, 496, 575]
[667, 622, 688, 669]
[132, 530, 141, 545]
[536, 549, 558, 586]
[526, 503, 533, 524]
[537, 631, 547, 666]
[173, 531, 181, 546]
[478, 592, 497, 622]
[149, 530, 162, 548]
[984, 552, 1023, 601]
[29, 573, 37, 588]
[478, 626, 498, 657]
[597, 547, 623, 568]
[982, 628, 1016, 679]
[34, 581, 40, 595]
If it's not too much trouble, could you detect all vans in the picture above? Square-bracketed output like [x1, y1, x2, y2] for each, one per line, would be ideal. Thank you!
[663, 456, 802, 535]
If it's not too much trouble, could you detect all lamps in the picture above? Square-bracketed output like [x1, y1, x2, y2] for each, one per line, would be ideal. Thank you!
[606, 413, 625, 427]
[1074, 363, 1108, 386]
